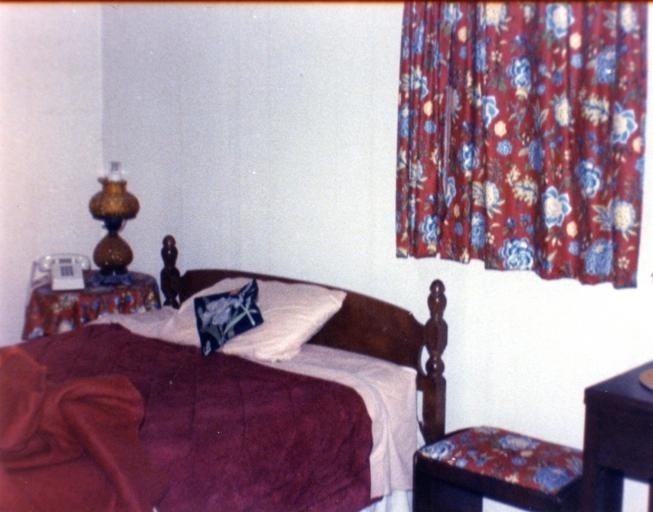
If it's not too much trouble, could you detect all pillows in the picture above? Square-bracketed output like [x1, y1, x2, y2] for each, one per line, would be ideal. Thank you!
[158, 276, 348, 365]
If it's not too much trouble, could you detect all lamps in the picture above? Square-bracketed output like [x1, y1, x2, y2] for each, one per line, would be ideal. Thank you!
[88, 160, 140, 287]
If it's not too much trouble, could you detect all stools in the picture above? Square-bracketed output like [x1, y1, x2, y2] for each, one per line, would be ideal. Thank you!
[413, 426, 597, 512]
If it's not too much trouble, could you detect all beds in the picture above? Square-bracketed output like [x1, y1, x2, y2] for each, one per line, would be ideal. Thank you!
[0, 234, 448, 512]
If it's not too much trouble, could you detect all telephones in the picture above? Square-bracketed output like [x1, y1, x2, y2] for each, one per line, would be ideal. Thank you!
[38, 253, 91, 291]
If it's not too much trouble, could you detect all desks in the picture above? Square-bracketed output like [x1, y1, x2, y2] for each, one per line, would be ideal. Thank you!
[584, 360, 652, 512]
[33, 270, 157, 338]
